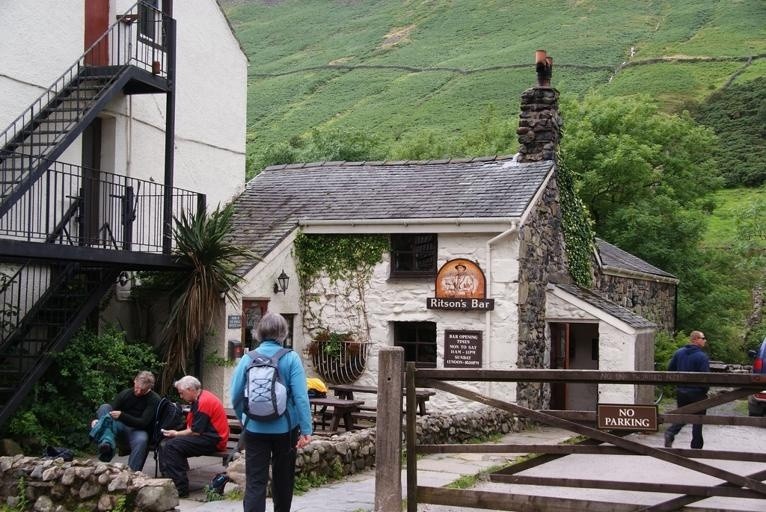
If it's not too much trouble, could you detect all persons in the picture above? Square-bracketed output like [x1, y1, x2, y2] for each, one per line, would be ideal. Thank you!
[158, 376, 230, 498]
[89, 370, 161, 472]
[231, 312, 313, 512]
[452, 262, 473, 290]
[664, 331, 711, 448]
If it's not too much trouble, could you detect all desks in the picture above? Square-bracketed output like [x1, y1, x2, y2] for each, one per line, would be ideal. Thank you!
[203, 382, 435, 466]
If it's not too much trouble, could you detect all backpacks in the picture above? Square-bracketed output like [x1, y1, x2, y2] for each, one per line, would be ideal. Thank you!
[152, 397, 184, 459]
[245, 347, 293, 422]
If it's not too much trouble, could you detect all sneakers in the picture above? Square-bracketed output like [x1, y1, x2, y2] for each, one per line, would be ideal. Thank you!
[665, 434, 672, 447]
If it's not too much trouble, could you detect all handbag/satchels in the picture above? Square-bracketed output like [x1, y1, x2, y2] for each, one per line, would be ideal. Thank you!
[306, 378, 328, 416]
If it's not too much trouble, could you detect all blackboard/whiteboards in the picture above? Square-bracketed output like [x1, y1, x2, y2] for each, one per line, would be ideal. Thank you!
[445, 329, 483, 368]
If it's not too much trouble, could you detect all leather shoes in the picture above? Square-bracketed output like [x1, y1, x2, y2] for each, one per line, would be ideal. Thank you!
[178, 489, 190, 499]
[99, 444, 112, 462]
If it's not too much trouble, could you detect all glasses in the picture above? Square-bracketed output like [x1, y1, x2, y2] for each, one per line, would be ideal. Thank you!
[698, 336, 706, 340]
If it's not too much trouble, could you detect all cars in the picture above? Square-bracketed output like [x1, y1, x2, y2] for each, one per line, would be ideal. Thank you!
[749, 338, 766, 417]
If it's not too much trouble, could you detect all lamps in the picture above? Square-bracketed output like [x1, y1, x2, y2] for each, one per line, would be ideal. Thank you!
[273, 268, 291, 297]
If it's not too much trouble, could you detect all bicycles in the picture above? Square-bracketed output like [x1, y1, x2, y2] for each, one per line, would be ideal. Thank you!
[655, 363, 664, 404]
